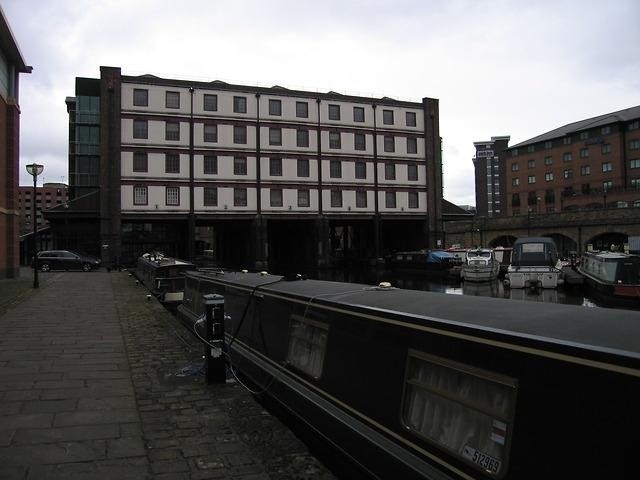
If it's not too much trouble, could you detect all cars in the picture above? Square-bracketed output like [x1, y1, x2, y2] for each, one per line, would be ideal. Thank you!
[31, 249, 103, 272]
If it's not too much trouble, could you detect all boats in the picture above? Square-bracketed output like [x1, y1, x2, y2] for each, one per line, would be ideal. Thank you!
[393, 235, 640, 297]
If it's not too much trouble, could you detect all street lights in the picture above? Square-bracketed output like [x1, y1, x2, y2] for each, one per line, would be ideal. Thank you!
[25, 162, 43, 292]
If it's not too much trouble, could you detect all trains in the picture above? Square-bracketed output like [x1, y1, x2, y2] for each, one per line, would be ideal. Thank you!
[137, 248, 640, 480]
